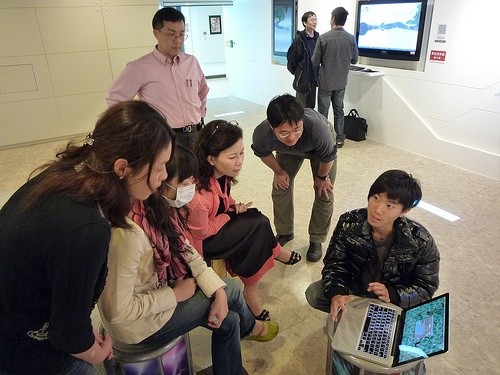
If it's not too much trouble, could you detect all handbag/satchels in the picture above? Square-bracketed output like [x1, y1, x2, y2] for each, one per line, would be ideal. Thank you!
[343, 108, 367, 142]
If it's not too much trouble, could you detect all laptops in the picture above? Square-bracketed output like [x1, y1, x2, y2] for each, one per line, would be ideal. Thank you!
[331, 292, 448, 367]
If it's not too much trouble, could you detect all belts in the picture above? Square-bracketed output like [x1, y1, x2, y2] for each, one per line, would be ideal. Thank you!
[170, 124, 200, 133]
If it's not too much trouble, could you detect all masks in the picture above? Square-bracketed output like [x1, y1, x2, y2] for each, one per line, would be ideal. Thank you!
[162, 183, 196, 209]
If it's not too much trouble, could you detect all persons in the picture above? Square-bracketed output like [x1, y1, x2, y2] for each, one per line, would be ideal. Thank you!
[311, 7, 359, 146]
[250, 94, 338, 262]
[0, 100, 300, 375]
[286, 11, 321, 111]
[305, 170, 440, 321]
[105, 7, 210, 151]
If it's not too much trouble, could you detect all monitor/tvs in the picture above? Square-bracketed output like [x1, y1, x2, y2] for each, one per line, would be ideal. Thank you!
[354, 0, 434, 72]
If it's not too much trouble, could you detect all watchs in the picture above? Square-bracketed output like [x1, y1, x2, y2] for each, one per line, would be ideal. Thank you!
[318, 173, 330, 181]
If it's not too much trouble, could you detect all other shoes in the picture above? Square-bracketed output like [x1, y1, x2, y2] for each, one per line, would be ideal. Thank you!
[307, 241, 322, 261]
[274, 251, 300, 265]
[275, 232, 294, 247]
[247, 302, 270, 321]
[239, 321, 279, 342]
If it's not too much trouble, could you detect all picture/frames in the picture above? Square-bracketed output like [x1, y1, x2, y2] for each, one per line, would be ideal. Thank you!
[208, 15, 222, 35]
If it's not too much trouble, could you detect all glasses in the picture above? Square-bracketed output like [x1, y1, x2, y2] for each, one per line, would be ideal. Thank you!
[272, 127, 304, 138]
[156, 28, 188, 40]
[206, 120, 239, 144]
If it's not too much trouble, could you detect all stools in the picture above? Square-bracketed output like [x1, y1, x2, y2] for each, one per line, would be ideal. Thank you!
[97, 320, 193, 375]
[325, 296, 426, 375]
